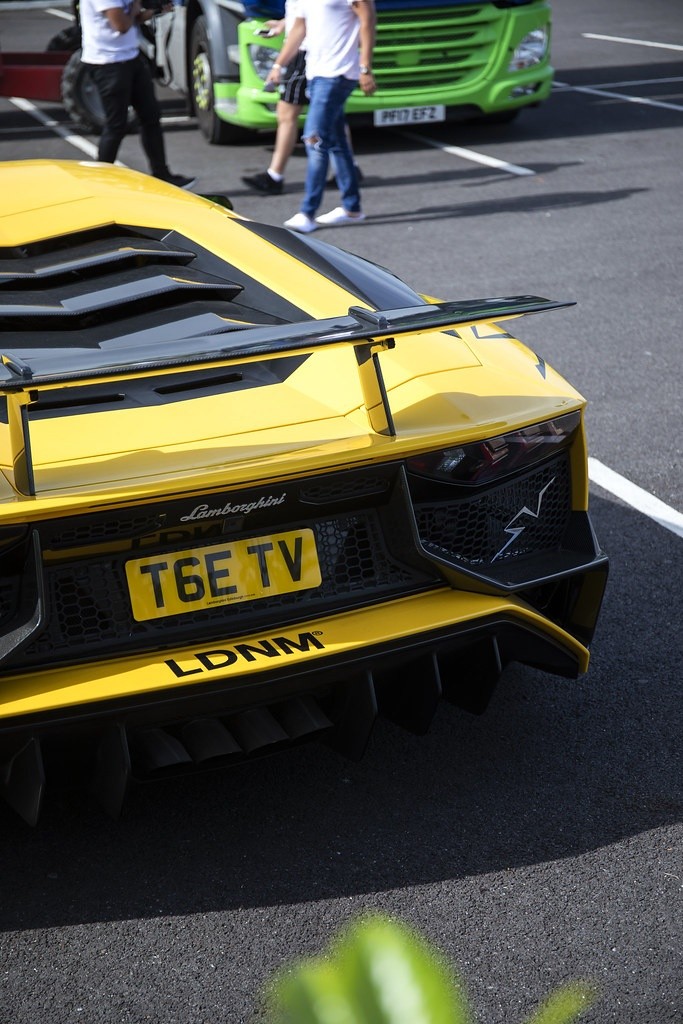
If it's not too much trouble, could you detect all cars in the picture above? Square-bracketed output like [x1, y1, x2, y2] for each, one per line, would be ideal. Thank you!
[0, 0, 144, 139]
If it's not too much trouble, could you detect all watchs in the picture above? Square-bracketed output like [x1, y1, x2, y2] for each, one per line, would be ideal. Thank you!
[358, 65, 372, 74]
[271, 63, 288, 75]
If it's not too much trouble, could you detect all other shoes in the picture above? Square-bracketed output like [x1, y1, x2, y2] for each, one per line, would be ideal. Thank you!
[240, 170, 285, 196]
[282, 212, 317, 233]
[161, 175, 199, 192]
[326, 162, 365, 190]
[314, 207, 366, 225]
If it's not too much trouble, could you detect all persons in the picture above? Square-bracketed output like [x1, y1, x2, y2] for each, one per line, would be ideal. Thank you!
[266, 0, 377, 234]
[239, 0, 363, 194]
[76, 0, 199, 191]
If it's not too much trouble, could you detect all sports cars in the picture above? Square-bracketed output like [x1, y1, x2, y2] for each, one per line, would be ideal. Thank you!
[0, 157, 609, 842]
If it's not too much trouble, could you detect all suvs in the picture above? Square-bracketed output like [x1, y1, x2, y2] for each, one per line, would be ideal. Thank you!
[146, 0, 555, 147]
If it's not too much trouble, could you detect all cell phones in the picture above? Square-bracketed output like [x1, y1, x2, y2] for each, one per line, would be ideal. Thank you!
[253, 28, 275, 36]
[264, 81, 278, 91]
